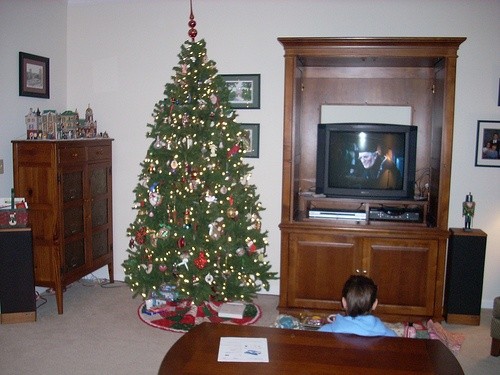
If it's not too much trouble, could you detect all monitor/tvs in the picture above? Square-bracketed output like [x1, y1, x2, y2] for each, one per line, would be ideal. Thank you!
[316, 123, 418, 201]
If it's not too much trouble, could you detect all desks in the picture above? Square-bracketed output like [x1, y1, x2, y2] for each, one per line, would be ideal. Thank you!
[157, 321, 465, 375]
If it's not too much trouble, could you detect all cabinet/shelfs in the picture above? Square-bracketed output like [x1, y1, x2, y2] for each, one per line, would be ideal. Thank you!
[11, 136, 114, 315]
[277, 36, 467, 323]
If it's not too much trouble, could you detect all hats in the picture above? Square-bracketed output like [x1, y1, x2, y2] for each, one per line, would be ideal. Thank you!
[355, 133, 380, 152]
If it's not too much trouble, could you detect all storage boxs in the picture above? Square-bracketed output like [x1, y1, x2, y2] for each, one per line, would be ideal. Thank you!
[0, 206, 29, 228]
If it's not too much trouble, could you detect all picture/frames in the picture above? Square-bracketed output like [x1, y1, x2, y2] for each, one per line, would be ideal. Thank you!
[475, 118, 500, 167]
[218, 73, 261, 109]
[237, 123, 260, 158]
[19, 51, 50, 99]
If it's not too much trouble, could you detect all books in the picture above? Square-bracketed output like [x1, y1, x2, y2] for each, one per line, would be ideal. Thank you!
[218, 303, 245, 319]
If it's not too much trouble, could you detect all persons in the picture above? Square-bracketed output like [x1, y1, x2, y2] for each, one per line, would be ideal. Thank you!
[483, 133, 500, 159]
[352, 133, 394, 181]
[318, 275, 399, 337]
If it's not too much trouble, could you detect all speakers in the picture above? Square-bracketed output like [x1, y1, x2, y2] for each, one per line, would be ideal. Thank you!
[0, 227, 37, 323]
[444, 228, 487, 326]
